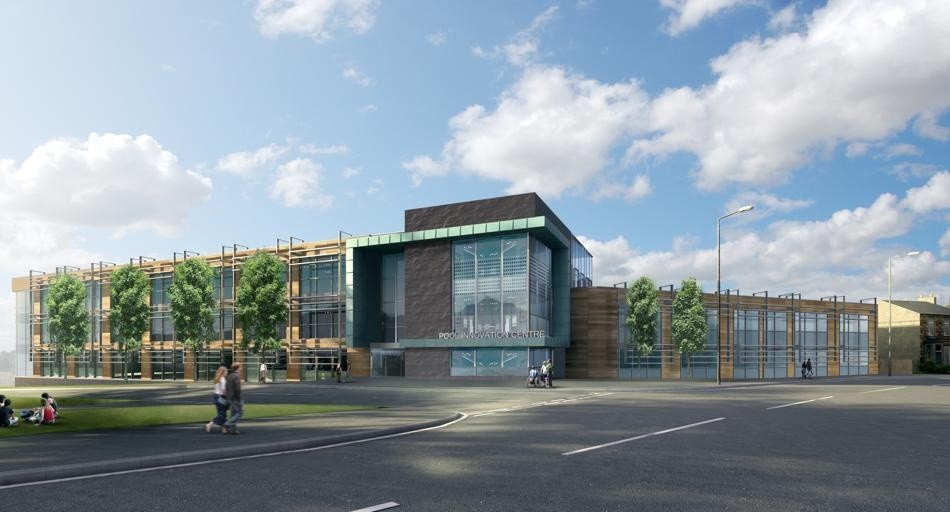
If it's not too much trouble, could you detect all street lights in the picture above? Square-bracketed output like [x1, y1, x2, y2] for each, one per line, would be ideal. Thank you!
[716, 205, 754, 385]
[887, 251, 920, 377]
